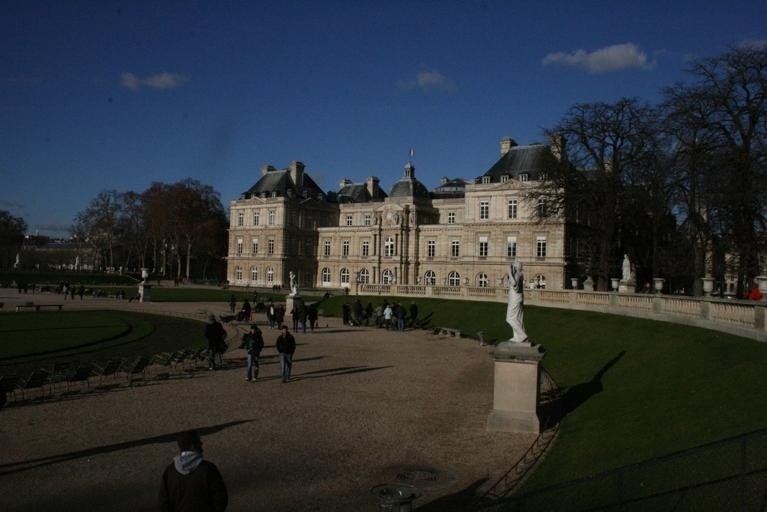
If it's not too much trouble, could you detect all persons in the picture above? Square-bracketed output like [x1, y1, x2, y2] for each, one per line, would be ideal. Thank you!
[230, 285, 418, 348]
[506, 261, 528, 344]
[204, 315, 228, 370]
[160, 430, 228, 512]
[623, 253, 631, 280]
[55, 276, 187, 303]
[244, 326, 262, 382]
[289, 271, 296, 294]
[277, 325, 295, 382]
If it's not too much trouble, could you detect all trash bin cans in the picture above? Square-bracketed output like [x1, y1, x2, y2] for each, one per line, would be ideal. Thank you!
[342, 303, 351, 324]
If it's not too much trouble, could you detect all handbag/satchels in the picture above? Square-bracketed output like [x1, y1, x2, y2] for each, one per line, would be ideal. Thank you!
[214, 341, 228, 354]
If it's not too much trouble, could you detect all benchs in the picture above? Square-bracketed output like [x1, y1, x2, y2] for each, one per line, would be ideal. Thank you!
[439, 327, 466, 339]
[15, 304, 65, 313]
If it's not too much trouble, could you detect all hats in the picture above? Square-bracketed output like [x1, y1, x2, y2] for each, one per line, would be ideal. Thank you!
[208, 315, 216, 322]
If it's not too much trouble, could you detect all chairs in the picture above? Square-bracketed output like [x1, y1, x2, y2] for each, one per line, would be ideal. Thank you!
[0, 345, 211, 408]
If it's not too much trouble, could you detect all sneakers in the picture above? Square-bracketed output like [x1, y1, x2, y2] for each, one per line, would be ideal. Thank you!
[246, 377, 258, 381]
